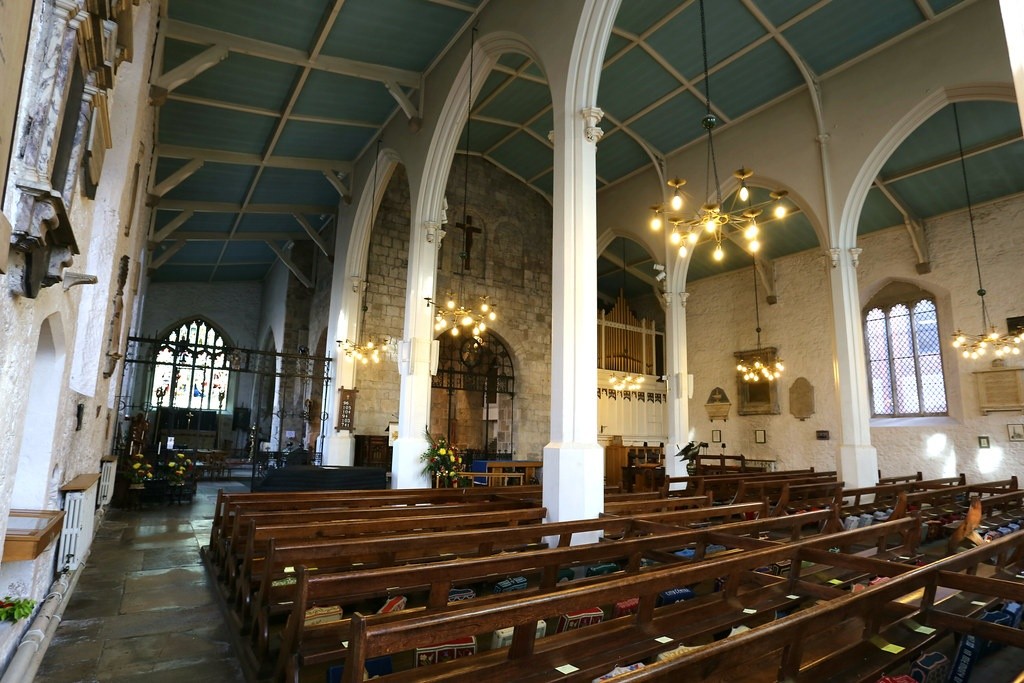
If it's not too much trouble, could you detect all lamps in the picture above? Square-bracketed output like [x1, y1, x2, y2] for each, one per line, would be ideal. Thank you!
[411, 0, 500, 344]
[641, 1, 789, 265]
[337, 153, 396, 365]
[946, 106, 1024, 358]
[733, 252, 785, 382]
[597, 251, 646, 394]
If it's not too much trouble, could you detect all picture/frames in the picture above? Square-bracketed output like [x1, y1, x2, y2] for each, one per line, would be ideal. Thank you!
[712, 430, 721, 442]
[1007, 422, 1023, 442]
[754, 429, 766, 444]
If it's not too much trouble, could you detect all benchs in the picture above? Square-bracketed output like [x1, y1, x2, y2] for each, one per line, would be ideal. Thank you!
[193, 453, 1024, 683]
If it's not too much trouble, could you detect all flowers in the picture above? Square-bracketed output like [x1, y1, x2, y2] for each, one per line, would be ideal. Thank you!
[166, 451, 197, 486]
[125, 453, 155, 484]
[423, 425, 464, 479]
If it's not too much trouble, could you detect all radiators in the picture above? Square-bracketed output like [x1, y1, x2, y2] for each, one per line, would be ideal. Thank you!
[96, 453, 117, 506]
[55, 469, 98, 572]
